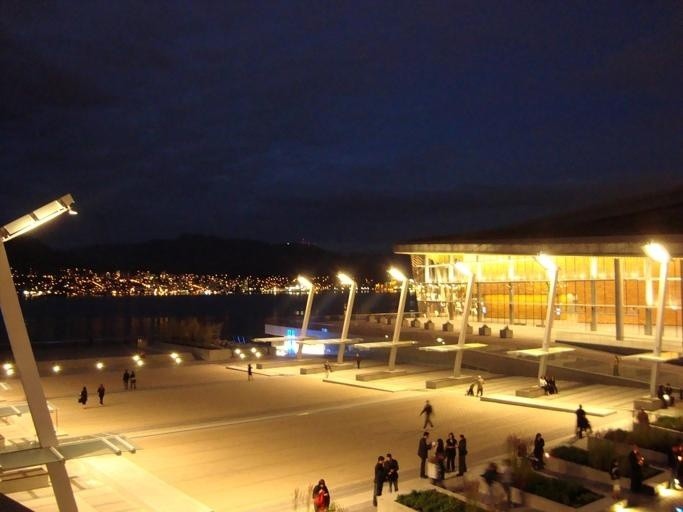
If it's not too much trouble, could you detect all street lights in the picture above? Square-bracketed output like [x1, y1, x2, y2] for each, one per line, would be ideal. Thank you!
[357, 265, 410, 380]
[515, 253, 559, 397]
[427, 262, 476, 389]
[299, 270, 356, 374]
[0, 192, 78, 512]
[630, 239, 679, 411]
[255, 273, 317, 369]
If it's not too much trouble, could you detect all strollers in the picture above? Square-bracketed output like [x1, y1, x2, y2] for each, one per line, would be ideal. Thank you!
[464, 383, 474, 397]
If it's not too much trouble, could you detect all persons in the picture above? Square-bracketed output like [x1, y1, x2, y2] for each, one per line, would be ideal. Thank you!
[629, 444, 642, 494]
[540, 375, 558, 395]
[576, 405, 586, 440]
[657, 381, 675, 409]
[637, 408, 649, 425]
[247, 363, 253, 380]
[482, 459, 515, 507]
[324, 360, 330, 378]
[467, 376, 485, 397]
[418, 432, 468, 491]
[532, 433, 545, 470]
[420, 400, 433, 429]
[372, 453, 399, 505]
[129, 370, 136, 389]
[96, 383, 106, 406]
[311, 478, 329, 511]
[668, 444, 683, 486]
[355, 353, 361, 369]
[80, 387, 86, 405]
[121, 368, 130, 389]
